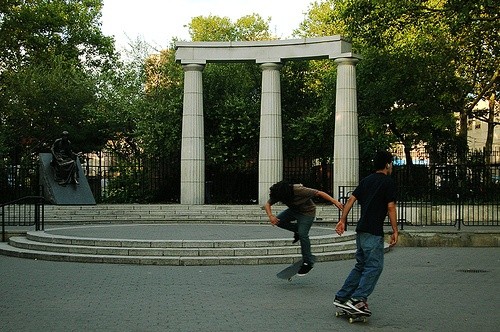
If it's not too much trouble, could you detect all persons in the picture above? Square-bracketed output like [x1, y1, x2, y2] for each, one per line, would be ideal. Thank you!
[332, 151, 399, 316]
[265, 179, 343, 277]
[50, 131, 86, 185]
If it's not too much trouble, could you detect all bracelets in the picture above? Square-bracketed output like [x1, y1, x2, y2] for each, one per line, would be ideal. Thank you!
[269, 215, 273, 218]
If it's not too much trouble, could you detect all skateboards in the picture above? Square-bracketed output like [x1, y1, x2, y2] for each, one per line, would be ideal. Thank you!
[335, 305, 371, 324]
[276, 255, 316, 282]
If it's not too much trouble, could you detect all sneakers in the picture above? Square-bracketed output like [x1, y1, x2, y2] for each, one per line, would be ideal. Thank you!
[346, 298, 372, 317]
[333, 295, 352, 310]
[292, 232, 300, 244]
[297, 263, 313, 277]
